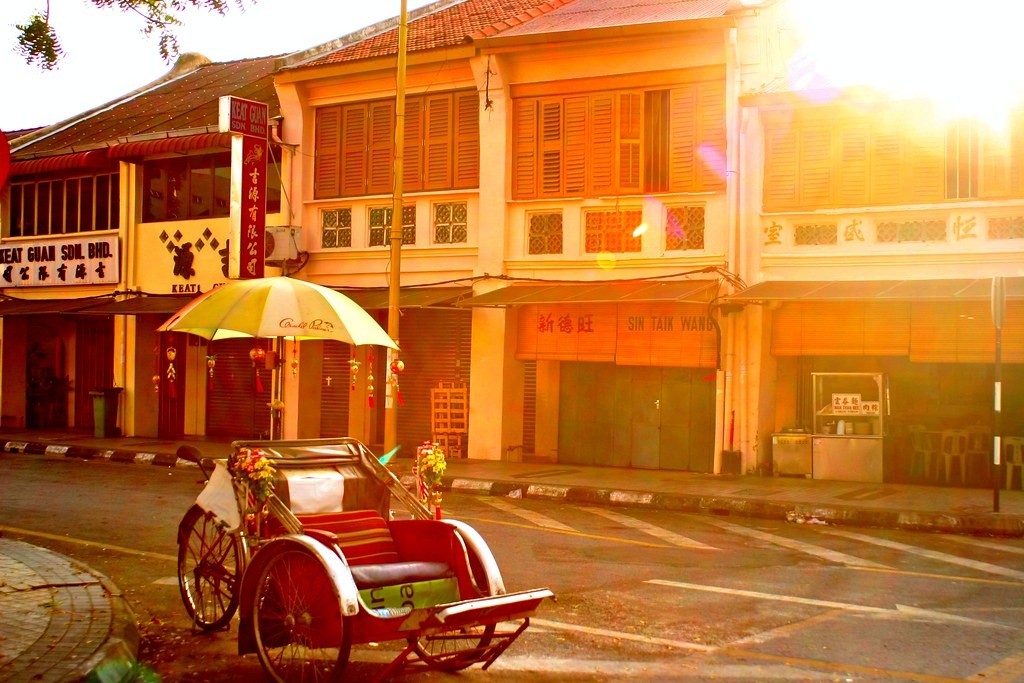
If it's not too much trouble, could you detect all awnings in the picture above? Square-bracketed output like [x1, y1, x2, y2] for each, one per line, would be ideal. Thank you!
[78, 297, 193, 315]
[339, 288, 473, 311]
[451, 280, 719, 308]
[724, 277, 1024, 300]
[0, 298, 114, 317]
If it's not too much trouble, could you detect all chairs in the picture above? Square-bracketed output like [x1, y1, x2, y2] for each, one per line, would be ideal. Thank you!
[907, 422, 1024, 493]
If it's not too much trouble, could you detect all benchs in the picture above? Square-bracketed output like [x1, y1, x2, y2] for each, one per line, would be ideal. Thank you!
[290, 509, 462, 610]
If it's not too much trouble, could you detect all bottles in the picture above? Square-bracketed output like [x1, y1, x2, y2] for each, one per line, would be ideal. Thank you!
[837, 420, 844, 436]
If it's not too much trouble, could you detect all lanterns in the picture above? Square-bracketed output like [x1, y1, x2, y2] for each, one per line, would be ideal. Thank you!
[390, 359, 404, 372]
[249, 347, 266, 361]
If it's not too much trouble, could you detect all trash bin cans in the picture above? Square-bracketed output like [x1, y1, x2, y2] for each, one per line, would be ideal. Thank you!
[89, 387, 123, 439]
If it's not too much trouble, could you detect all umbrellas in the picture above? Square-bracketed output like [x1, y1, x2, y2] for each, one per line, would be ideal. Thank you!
[156, 275, 402, 441]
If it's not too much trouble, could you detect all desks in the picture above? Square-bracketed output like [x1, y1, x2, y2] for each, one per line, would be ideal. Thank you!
[919, 430, 944, 475]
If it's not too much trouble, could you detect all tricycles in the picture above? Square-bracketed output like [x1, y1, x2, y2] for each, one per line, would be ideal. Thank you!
[175, 436, 555, 682]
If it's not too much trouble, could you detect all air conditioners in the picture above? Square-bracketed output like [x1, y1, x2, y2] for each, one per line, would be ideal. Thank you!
[263, 223, 302, 261]
